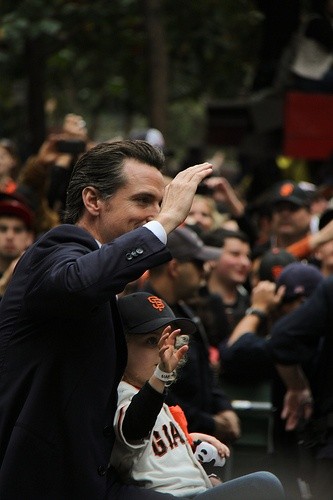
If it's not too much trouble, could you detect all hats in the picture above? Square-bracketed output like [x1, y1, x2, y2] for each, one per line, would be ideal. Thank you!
[165, 225, 222, 261]
[0, 191, 35, 224]
[272, 260, 325, 301]
[265, 180, 309, 209]
[258, 247, 297, 282]
[118, 291, 199, 335]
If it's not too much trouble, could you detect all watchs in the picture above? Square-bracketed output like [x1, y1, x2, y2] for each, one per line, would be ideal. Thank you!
[245, 308, 269, 319]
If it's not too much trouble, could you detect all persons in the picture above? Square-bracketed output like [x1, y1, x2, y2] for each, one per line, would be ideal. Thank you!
[109, 291, 289, 500]
[0, 138, 224, 500]
[0, 112, 333, 500]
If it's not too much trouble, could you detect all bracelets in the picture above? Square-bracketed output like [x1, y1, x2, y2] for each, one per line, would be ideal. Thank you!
[153, 365, 178, 387]
